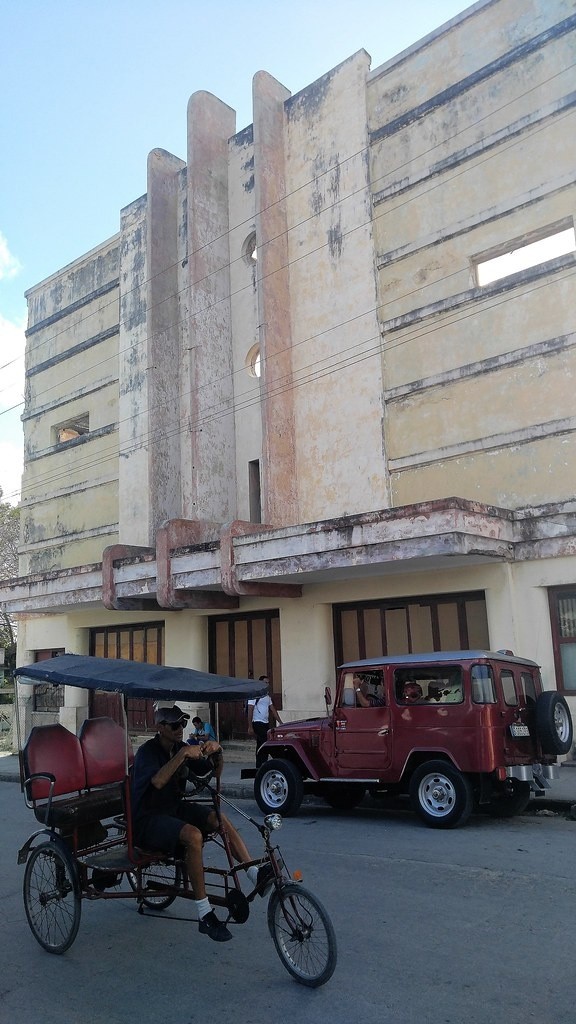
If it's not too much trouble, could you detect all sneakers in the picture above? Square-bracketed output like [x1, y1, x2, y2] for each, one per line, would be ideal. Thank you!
[257, 859, 285, 898]
[199, 907, 233, 942]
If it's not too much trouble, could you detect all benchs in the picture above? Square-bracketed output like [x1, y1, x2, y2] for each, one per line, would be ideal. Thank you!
[23, 716, 136, 831]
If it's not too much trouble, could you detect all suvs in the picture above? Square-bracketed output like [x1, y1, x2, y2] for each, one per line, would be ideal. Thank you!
[240, 648, 574, 831]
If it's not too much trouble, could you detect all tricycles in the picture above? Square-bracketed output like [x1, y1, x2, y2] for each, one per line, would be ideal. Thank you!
[11, 653, 339, 989]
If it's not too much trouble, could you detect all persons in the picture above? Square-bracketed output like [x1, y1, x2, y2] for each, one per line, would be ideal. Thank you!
[131, 708, 273, 942]
[248, 675, 283, 769]
[352, 677, 386, 708]
[187, 717, 215, 745]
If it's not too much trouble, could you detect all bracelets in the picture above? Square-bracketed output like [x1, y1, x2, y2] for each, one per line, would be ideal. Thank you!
[355, 688, 361, 692]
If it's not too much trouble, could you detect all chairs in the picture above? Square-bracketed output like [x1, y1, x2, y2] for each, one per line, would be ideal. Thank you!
[402, 682, 424, 705]
[425, 681, 446, 704]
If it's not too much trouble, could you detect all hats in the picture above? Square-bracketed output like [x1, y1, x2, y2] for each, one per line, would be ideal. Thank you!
[155, 705, 190, 724]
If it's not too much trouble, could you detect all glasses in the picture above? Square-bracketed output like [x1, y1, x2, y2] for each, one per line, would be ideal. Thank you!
[165, 720, 188, 730]
